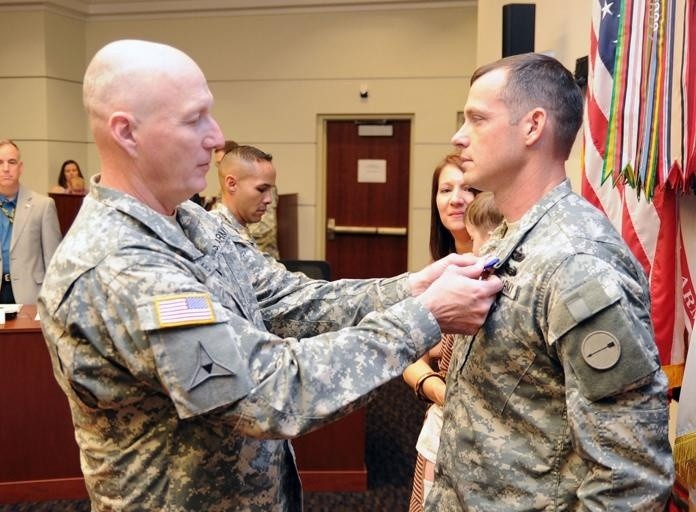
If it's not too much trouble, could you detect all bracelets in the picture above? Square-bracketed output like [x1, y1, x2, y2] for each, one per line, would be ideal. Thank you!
[415, 374, 446, 404]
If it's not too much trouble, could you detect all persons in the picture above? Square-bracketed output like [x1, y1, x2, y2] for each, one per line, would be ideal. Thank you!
[423, 52, 675, 512]
[210, 146, 277, 251]
[0, 143, 61, 307]
[414, 191, 505, 507]
[39, 38, 505, 512]
[209, 141, 280, 262]
[402, 155, 483, 512]
[52, 160, 91, 195]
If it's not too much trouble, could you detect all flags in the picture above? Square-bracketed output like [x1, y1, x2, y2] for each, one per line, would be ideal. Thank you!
[581, 0, 696, 402]
